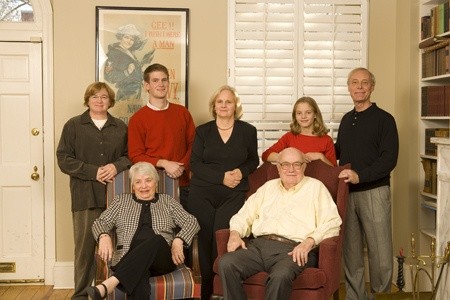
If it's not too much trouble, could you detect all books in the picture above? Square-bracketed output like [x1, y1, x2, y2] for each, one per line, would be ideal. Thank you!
[421, 86, 450, 117]
[419, 38, 450, 78]
[424, 160, 437, 194]
[421, 2, 450, 39]
[425, 127, 449, 156]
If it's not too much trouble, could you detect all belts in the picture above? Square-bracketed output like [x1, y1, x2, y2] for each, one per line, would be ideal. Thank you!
[262, 235, 297, 245]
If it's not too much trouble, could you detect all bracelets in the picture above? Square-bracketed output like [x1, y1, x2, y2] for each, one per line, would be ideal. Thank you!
[99, 235, 108, 238]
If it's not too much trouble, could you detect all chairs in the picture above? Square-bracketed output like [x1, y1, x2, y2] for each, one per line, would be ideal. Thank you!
[94, 168, 202, 300]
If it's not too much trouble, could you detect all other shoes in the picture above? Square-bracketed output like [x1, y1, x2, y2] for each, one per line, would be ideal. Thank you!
[87, 283, 107, 300]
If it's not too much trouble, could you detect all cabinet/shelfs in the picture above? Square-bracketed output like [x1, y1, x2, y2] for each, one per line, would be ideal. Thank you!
[419, 28, 450, 257]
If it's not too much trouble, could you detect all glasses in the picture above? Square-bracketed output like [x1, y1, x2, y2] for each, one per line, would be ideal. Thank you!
[279, 161, 304, 170]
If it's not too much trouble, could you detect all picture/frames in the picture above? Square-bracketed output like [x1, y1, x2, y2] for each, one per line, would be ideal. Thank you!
[94, 5, 191, 124]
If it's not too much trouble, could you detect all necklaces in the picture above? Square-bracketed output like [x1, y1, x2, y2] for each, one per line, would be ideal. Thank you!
[217, 125, 233, 130]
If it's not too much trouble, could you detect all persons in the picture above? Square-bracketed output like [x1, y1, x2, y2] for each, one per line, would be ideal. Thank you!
[218, 147, 342, 300]
[57, 81, 131, 300]
[86, 162, 201, 300]
[186, 86, 259, 300]
[262, 97, 337, 168]
[334, 67, 400, 300]
[127, 63, 195, 268]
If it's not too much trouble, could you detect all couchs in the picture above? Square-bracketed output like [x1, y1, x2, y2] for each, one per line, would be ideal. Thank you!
[212, 158, 351, 300]
